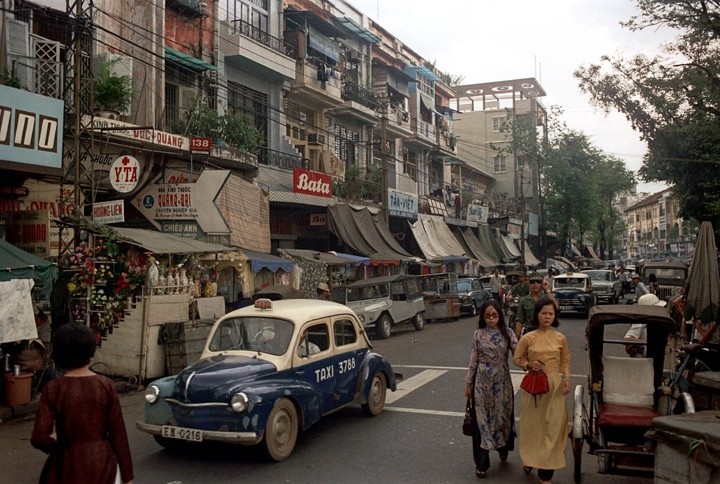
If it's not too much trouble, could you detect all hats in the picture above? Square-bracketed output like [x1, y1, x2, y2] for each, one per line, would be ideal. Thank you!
[631, 274, 641, 279]
[639, 293, 667, 307]
[529, 274, 544, 280]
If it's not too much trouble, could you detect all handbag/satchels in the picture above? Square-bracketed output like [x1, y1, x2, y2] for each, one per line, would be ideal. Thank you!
[462, 396, 474, 435]
[520, 366, 551, 395]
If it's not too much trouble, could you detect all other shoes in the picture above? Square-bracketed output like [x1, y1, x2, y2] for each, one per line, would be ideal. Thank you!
[476, 469, 486, 477]
[525, 464, 533, 473]
[499, 454, 507, 466]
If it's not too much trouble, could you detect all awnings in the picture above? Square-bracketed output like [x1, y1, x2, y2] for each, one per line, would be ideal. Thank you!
[336, 16, 379, 42]
[164, 46, 218, 71]
[101, 226, 470, 275]
[386, 65, 462, 118]
[285, 8, 350, 41]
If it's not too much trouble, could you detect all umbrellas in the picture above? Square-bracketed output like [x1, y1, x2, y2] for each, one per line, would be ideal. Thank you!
[682, 222, 720, 319]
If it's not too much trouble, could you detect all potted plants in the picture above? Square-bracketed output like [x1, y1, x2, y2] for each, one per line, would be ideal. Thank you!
[91, 55, 134, 119]
[347, 166, 366, 200]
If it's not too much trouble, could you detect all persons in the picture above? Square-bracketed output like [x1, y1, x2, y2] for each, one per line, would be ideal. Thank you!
[297, 328, 320, 357]
[512, 298, 572, 484]
[607, 264, 627, 283]
[623, 294, 667, 358]
[675, 304, 720, 375]
[490, 270, 502, 305]
[314, 283, 329, 300]
[31, 322, 134, 483]
[674, 289, 687, 342]
[632, 274, 659, 303]
[506, 269, 556, 339]
[464, 301, 528, 479]
[35, 312, 50, 342]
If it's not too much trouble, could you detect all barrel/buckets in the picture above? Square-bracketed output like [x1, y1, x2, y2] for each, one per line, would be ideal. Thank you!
[3, 372, 34, 405]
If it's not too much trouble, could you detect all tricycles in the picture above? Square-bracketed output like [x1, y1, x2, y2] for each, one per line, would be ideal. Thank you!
[570, 304, 697, 484]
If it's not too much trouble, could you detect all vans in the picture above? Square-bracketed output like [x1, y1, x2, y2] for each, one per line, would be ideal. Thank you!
[635, 257, 690, 302]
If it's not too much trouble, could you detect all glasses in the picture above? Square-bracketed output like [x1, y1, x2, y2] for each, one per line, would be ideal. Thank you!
[529, 280, 542, 284]
[484, 313, 499, 318]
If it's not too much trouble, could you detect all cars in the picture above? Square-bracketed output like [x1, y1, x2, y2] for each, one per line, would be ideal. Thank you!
[137, 297, 404, 463]
[343, 274, 427, 339]
[418, 271, 527, 323]
[550, 269, 624, 317]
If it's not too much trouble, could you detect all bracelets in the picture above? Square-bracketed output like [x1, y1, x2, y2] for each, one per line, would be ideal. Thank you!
[546, 290, 551, 293]
